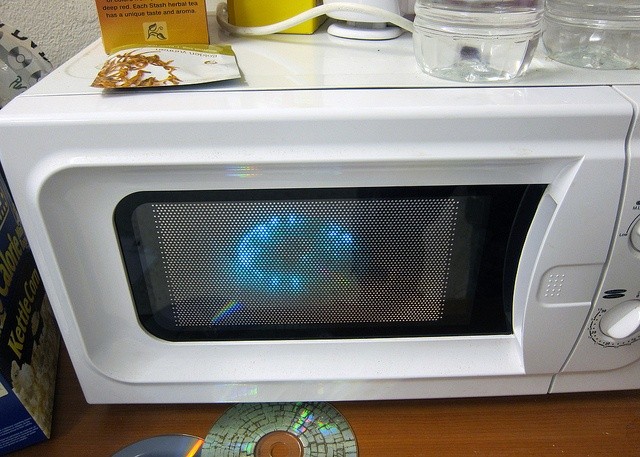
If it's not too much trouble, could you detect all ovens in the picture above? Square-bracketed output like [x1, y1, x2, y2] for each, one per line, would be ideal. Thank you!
[0, 18, 640, 404]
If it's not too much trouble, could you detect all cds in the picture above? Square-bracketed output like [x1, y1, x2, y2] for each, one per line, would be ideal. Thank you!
[200, 402, 358, 456]
